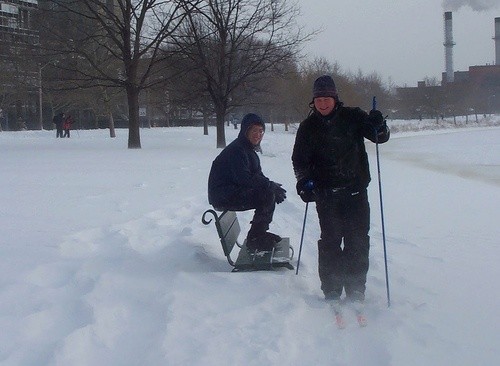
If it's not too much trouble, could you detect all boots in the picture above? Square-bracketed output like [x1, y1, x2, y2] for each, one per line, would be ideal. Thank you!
[245, 221, 282, 251]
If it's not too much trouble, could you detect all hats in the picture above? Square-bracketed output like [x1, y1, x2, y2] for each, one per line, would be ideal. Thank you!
[241, 113, 265, 135]
[312, 74, 339, 98]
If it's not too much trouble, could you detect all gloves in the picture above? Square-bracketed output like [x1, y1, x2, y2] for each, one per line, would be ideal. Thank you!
[368, 110, 383, 125]
[269, 181, 287, 204]
[297, 177, 319, 202]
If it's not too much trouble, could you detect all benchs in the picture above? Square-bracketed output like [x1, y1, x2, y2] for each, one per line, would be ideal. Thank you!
[201, 209, 294, 271]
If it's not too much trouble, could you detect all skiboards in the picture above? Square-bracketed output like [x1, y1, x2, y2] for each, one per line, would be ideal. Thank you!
[331, 298, 368, 329]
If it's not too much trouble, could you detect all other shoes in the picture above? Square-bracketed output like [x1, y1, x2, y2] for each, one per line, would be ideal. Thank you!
[325, 289, 341, 300]
[347, 289, 366, 302]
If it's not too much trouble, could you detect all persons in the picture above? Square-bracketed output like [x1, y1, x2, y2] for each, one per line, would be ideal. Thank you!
[208, 113, 287, 249]
[52, 113, 74, 138]
[291, 74, 391, 301]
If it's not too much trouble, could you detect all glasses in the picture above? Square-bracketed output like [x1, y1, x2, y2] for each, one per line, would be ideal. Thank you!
[248, 127, 265, 135]
